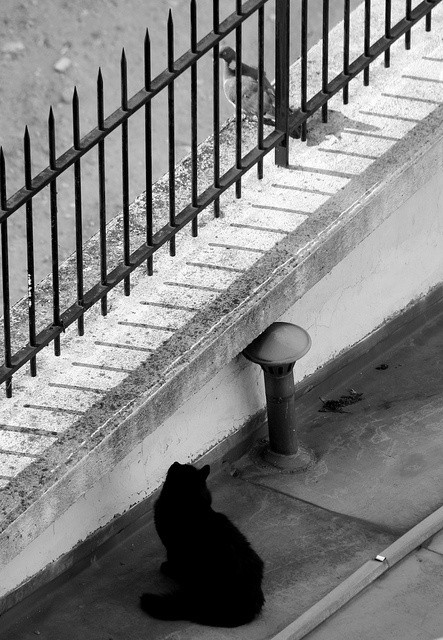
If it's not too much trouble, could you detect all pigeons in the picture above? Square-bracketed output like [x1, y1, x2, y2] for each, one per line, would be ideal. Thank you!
[219, 46, 301, 138]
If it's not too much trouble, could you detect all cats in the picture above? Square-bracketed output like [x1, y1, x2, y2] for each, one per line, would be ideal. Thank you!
[138, 461, 264, 628]
